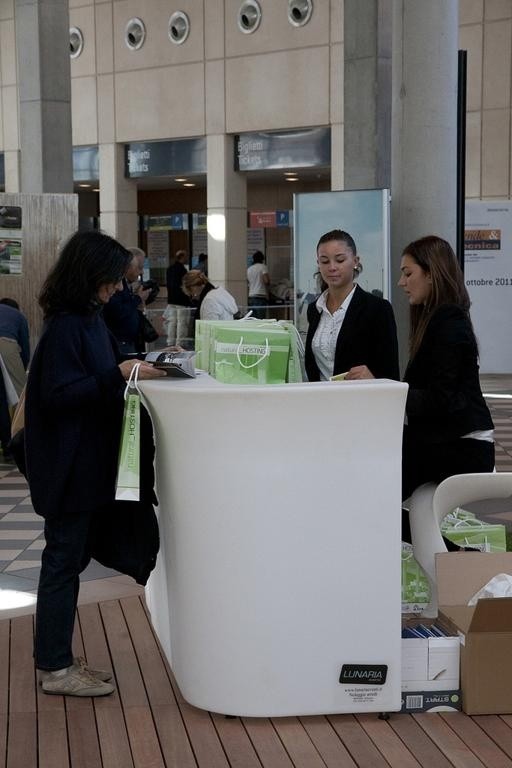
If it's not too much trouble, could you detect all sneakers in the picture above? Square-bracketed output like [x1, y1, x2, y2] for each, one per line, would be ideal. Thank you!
[37, 663, 115, 698]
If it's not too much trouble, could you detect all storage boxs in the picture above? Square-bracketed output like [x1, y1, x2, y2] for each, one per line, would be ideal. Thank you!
[432, 551, 512, 717]
[401, 618, 462, 715]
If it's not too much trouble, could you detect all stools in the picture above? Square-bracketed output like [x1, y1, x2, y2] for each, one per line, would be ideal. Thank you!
[407, 471, 512, 585]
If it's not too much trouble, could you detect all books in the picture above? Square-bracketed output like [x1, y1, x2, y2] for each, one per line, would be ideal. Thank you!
[142, 349, 202, 378]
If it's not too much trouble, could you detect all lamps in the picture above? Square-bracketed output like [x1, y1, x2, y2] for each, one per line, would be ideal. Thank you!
[285, 0, 314, 27]
[235, 0, 264, 35]
[166, 10, 193, 47]
[68, 25, 84, 60]
[122, 16, 147, 52]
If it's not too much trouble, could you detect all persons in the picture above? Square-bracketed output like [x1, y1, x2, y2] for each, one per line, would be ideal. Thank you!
[304, 229, 400, 383]
[11, 228, 168, 697]
[163, 247, 270, 344]
[0, 298, 30, 461]
[345, 236, 496, 551]
[100, 246, 159, 360]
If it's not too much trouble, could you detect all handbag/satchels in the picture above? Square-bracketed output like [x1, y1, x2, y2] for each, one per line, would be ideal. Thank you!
[440, 507, 507, 553]
[403, 544, 433, 613]
[194, 318, 303, 385]
[115, 359, 141, 503]
